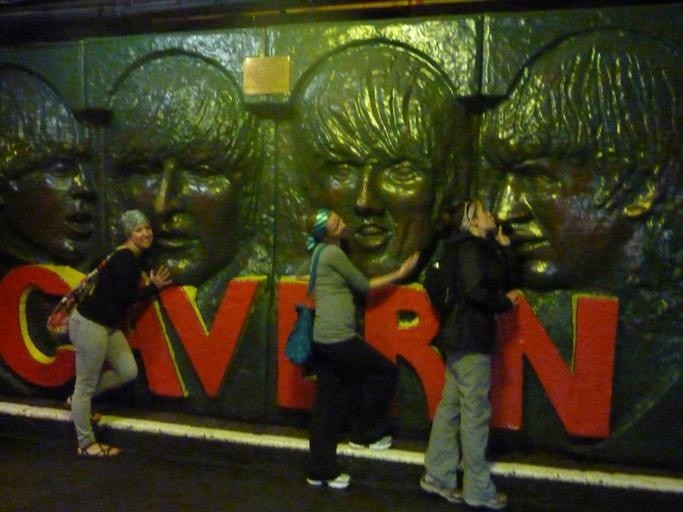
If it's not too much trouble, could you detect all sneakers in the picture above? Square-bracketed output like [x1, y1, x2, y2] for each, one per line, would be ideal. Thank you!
[419, 472, 510, 510]
[348, 435, 393, 450]
[307, 472, 352, 489]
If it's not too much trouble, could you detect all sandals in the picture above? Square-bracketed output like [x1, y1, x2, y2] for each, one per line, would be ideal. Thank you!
[63, 395, 102, 423]
[75, 442, 122, 460]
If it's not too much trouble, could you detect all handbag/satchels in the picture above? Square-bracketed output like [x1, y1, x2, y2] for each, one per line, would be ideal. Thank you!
[46, 279, 94, 343]
[284, 303, 315, 366]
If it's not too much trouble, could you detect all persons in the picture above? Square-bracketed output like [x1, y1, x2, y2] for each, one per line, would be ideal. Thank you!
[0, 57, 108, 271]
[291, 32, 466, 281]
[480, 23, 682, 292]
[298, 205, 422, 487]
[107, 51, 256, 285]
[64, 208, 169, 458]
[408, 199, 525, 509]
